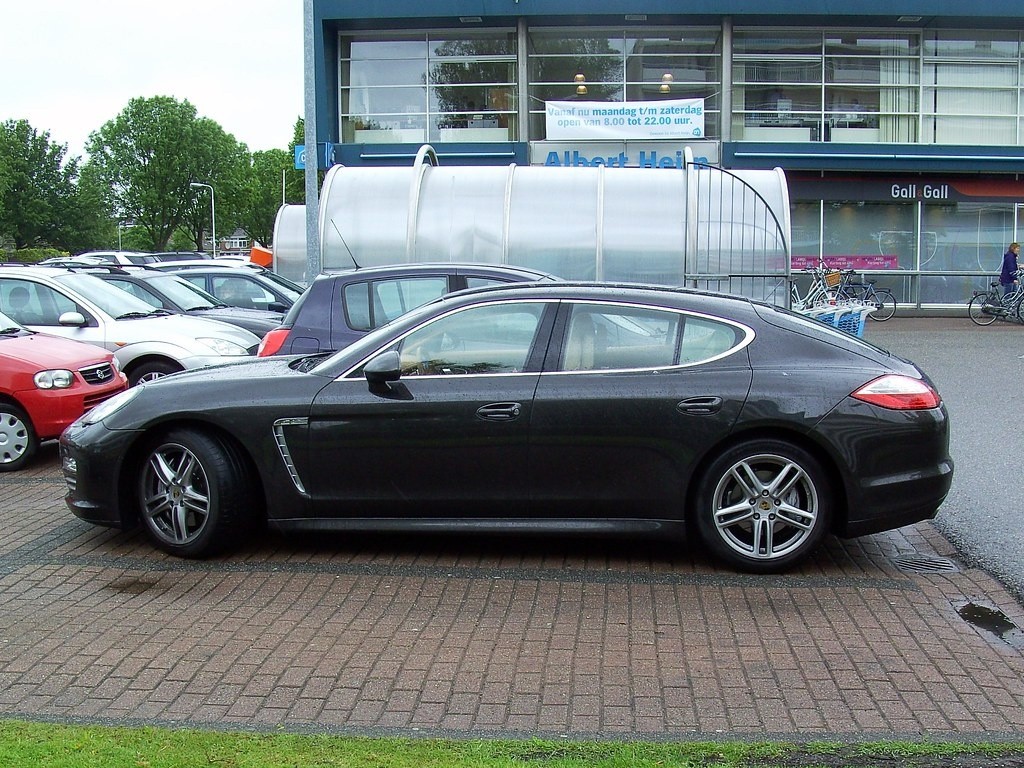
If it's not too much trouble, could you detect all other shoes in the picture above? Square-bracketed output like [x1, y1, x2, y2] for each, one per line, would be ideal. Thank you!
[1002, 318, 1013, 322]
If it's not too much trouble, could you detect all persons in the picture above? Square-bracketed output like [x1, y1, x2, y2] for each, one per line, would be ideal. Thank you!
[999, 243, 1024, 317]
[850, 99, 864, 111]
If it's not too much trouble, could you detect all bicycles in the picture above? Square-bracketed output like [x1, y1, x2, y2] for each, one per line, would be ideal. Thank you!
[967, 269, 1024, 326]
[791, 257, 896, 322]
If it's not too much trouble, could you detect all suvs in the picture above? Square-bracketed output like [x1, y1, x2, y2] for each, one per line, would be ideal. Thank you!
[0, 264, 287, 341]
[62, 250, 164, 271]
[37, 257, 117, 269]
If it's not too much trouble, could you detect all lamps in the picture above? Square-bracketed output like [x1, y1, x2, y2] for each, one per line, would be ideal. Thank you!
[573, 66, 586, 81]
[577, 86, 587, 94]
[659, 85, 671, 94]
[662, 70, 674, 81]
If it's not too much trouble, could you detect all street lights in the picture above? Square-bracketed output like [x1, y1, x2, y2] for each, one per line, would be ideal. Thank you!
[191, 183, 216, 259]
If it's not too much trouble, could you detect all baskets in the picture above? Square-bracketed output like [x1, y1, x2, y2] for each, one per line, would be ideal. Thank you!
[816, 306, 877, 339]
[825, 271, 841, 287]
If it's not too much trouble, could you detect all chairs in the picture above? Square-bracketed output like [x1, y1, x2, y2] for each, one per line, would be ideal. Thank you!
[564, 313, 595, 370]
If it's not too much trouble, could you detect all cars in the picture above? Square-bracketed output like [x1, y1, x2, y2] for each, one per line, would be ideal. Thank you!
[257, 263, 660, 357]
[0, 266, 261, 390]
[122, 268, 300, 314]
[58, 283, 954, 578]
[140, 256, 307, 303]
[0, 310, 128, 472]
[154, 252, 213, 261]
